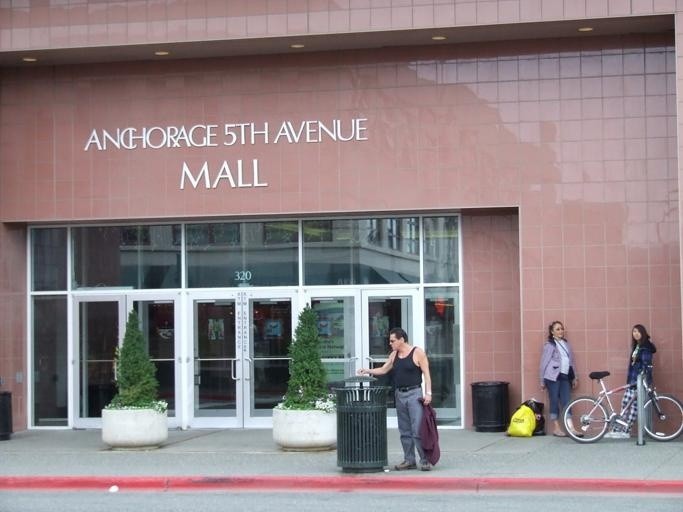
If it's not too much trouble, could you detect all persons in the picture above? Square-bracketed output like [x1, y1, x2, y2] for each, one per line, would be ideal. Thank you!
[540, 321, 584, 437]
[604, 324, 656, 438]
[358, 328, 432, 471]
[375, 310, 389, 336]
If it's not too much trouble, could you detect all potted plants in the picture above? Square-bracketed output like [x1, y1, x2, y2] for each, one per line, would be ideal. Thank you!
[272, 303, 338, 451]
[101, 310, 168, 452]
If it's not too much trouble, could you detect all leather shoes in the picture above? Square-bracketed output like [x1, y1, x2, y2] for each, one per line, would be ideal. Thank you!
[550, 428, 567, 437]
[570, 430, 584, 438]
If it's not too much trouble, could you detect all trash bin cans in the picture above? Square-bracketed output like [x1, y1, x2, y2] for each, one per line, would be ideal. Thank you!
[331, 376, 392, 473]
[471, 381, 510, 432]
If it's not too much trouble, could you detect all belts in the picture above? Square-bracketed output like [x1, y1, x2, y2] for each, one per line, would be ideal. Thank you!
[397, 384, 422, 392]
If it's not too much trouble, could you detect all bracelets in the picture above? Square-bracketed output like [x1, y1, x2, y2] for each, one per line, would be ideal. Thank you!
[424, 391, 432, 395]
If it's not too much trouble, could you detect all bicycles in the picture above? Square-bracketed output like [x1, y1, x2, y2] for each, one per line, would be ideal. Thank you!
[560, 365, 683, 443]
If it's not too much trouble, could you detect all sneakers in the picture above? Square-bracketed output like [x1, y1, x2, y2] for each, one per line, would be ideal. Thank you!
[420, 460, 432, 472]
[603, 430, 631, 439]
[393, 460, 417, 471]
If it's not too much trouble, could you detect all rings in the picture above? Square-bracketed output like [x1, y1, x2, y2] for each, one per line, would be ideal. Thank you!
[359, 369, 363, 372]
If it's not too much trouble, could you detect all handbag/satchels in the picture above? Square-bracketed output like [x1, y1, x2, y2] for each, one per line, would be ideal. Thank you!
[516, 400, 545, 435]
[508, 405, 536, 437]
[568, 365, 576, 380]
